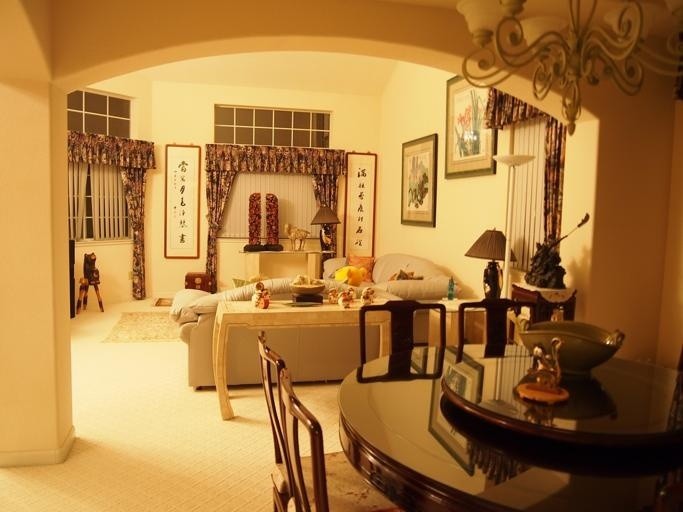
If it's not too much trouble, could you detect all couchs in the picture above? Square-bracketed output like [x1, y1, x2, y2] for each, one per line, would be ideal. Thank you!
[322, 252, 462, 345]
[170, 277, 404, 390]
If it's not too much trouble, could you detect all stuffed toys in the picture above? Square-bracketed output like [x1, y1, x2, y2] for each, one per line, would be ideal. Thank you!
[253, 283, 269, 308]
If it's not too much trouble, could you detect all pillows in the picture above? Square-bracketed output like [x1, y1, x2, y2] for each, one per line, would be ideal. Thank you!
[231, 278, 259, 288]
[346, 252, 374, 282]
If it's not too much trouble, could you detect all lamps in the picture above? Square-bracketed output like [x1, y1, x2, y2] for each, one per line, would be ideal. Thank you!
[454, 0, 683, 134]
[464, 228, 517, 299]
[310, 202, 341, 248]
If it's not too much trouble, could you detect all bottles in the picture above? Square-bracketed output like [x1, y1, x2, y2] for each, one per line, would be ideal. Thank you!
[447, 275, 454, 300]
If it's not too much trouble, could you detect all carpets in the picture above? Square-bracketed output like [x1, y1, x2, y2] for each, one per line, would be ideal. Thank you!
[102, 310, 181, 342]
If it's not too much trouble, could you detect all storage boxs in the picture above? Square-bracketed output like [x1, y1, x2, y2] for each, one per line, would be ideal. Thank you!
[185, 272, 217, 294]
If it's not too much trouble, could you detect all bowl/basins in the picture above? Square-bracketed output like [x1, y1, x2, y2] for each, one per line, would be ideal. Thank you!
[515, 320, 625, 377]
[512, 371, 620, 426]
[289, 282, 324, 296]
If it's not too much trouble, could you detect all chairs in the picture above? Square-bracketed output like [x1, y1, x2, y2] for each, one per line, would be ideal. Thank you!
[359, 298, 447, 364]
[254, 329, 407, 512]
[456, 298, 540, 363]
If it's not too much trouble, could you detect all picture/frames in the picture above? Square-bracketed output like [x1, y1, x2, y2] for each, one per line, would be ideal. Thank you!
[444, 75, 497, 181]
[428, 343, 485, 476]
[165, 144, 200, 260]
[401, 133, 438, 227]
[343, 152, 377, 257]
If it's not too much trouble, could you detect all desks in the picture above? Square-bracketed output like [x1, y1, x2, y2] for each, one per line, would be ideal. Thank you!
[508, 283, 577, 344]
[236, 249, 335, 282]
[211, 297, 416, 421]
[337, 344, 682, 512]
[429, 303, 484, 348]
